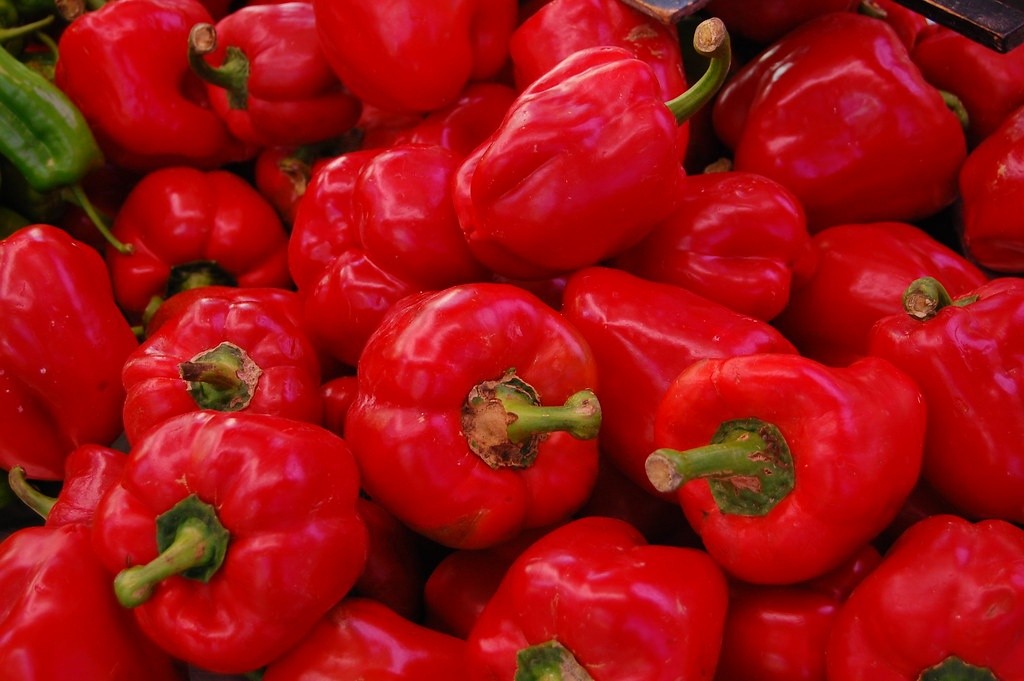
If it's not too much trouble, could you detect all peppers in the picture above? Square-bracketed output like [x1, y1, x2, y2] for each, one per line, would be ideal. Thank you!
[0, 0, 1024, 681]
